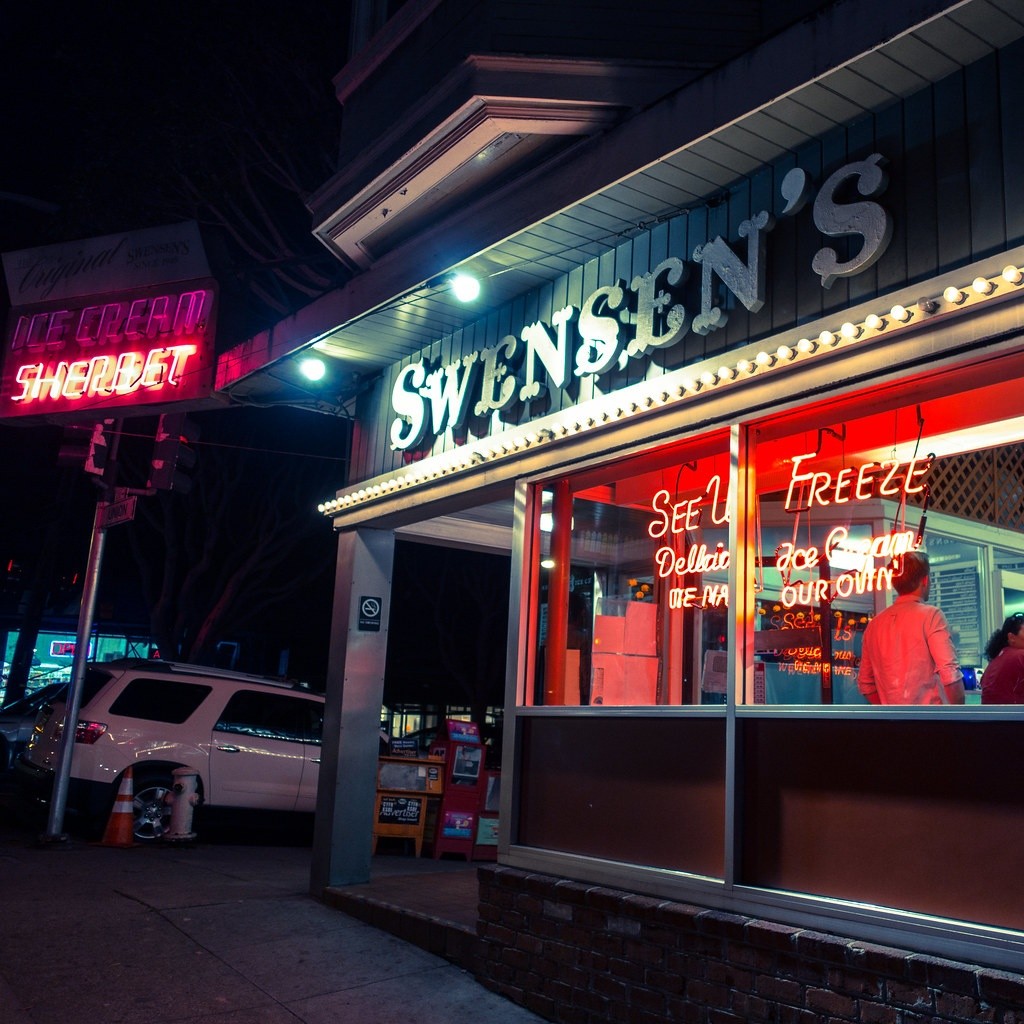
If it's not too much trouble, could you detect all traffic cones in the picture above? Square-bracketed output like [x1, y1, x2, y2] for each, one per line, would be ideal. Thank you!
[88, 766, 143, 849]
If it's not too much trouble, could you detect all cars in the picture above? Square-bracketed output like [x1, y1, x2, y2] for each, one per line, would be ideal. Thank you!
[0, 680, 70, 778]
[405, 727, 498, 773]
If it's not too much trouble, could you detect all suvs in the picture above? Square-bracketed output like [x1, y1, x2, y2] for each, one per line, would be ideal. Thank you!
[18, 656, 402, 840]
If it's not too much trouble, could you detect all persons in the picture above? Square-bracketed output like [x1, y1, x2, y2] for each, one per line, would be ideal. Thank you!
[858, 551, 965, 705]
[980, 612, 1024, 704]
